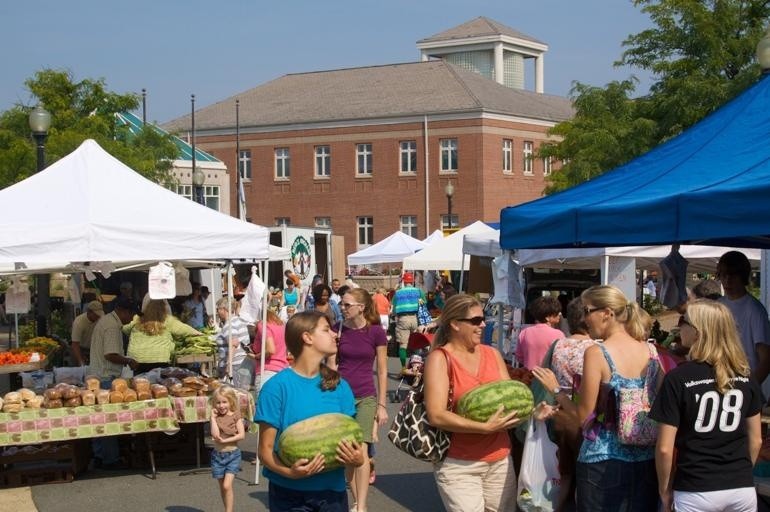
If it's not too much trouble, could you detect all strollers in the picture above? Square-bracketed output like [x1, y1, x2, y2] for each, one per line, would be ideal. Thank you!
[392, 327, 439, 401]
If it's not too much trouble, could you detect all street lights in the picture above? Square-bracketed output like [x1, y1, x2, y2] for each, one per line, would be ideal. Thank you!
[753, 16, 770, 74]
[444, 178, 455, 231]
[190, 169, 204, 205]
[29, 105, 51, 336]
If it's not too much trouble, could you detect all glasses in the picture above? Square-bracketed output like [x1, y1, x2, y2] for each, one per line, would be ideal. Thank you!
[340, 302, 361, 308]
[457, 315, 486, 326]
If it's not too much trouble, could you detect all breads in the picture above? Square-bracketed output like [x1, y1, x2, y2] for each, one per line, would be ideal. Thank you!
[0, 376, 219, 413]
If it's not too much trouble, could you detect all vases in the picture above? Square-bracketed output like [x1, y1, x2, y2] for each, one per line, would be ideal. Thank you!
[0, 358, 49, 374]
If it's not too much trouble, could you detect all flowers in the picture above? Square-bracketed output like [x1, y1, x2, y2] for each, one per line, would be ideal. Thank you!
[25, 337, 61, 369]
[0, 350, 47, 365]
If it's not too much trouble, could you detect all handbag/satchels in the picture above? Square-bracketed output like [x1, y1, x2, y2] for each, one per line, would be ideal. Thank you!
[387, 389, 455, 465]
[618, 386, 662, 448]
[417, 297, 433, 326]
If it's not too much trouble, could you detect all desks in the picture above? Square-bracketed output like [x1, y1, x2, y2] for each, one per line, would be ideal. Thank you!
[2, 390, 174, 482]
[162, 378, 258, 478]
[174, 352, 215, 379]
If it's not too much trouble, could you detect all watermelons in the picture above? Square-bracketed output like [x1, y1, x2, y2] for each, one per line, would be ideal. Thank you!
[278, 413, 365, 473]
[456, 380, 534, 428]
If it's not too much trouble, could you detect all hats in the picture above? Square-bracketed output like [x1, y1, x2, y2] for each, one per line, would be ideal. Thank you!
[401, 273, 416, 283]
[114, 294, 145, 317]
[87, 300, 106, 317]
